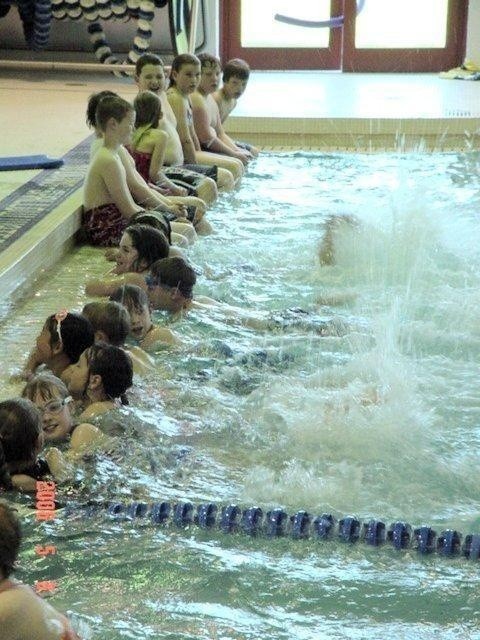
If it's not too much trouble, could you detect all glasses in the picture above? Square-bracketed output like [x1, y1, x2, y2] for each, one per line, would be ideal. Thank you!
[39, 398, 71, 415]
[55, 309, 68, 340]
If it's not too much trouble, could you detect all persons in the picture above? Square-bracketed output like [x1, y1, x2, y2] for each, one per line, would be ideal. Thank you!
[83, 54, 258, 247]
[145, 216, 358, 334]
[0, 503, 84, 639]
[1, 310, 133, 494]
[82, 210, 212, 375]
